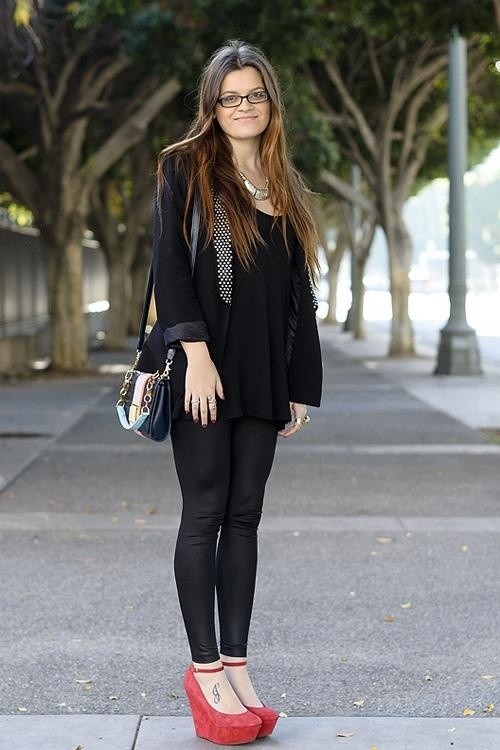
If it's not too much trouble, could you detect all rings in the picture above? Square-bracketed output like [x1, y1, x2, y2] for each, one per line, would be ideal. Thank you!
[207, 396, 217, 410]
[295, 418, 303, 427]
[190, 400, 198, 404]
[304, 416, 310, 425]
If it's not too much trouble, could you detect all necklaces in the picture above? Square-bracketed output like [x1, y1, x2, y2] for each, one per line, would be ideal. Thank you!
[236, 169, 271, 200]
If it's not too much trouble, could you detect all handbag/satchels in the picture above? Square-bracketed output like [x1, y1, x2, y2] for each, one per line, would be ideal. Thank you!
[117, 367, 172, 443]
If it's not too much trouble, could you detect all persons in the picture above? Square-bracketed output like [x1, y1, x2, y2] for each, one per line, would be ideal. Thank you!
[151, 41, 324, 745]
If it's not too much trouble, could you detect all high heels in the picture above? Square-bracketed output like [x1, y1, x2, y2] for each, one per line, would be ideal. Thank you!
[184, 662, 263, 746]
[222, 660, 278, 739]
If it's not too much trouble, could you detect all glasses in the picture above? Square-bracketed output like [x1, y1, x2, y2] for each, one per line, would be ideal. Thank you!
[217, 90, 270, 108]
